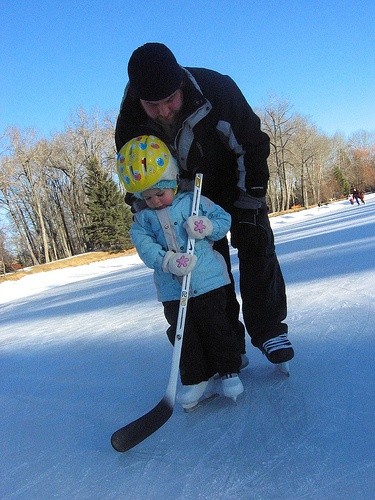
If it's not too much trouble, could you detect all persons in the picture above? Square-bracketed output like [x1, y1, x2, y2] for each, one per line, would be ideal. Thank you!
[349, 187, 365, 206]
[114, 43, 294, 378]
[117, 136, 243, 408]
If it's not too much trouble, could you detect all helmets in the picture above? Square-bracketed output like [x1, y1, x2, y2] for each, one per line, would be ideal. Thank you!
[117, 136, 170, 193]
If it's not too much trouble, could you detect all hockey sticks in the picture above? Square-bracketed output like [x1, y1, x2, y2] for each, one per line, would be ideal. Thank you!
[110, 174, 204, 453]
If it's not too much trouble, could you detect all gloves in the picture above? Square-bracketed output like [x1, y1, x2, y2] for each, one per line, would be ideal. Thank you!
[231, 191, 268, 247]
[163, 251, 197, 276]
[183, 216, 213, 240]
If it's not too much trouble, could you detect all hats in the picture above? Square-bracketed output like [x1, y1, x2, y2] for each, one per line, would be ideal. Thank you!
[128, 43, 184, 101]
[134, 181, 177, 199]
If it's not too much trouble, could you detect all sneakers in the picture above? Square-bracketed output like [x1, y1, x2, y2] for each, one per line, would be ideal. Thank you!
[260, 335, 294, 377]
[176, 380, 219, 413]
[222, 354, 249, 404]
